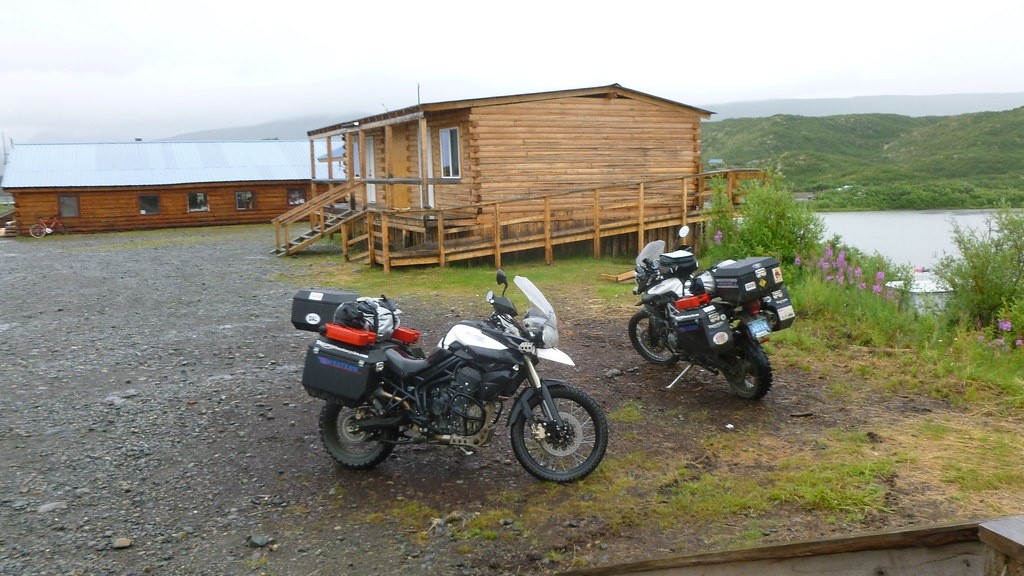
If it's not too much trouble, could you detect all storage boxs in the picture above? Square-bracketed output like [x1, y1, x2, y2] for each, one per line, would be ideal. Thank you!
[758, 287, 797, 333]
[290, 285, 361, 334]
[673, 302, 735, 359]
[713, 257, 786, 306]
[300, 337, 389, 409]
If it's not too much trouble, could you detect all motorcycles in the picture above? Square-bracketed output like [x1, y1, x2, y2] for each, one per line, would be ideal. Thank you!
[628, 224, 797, 403]
[292, 269, 609, 485]
